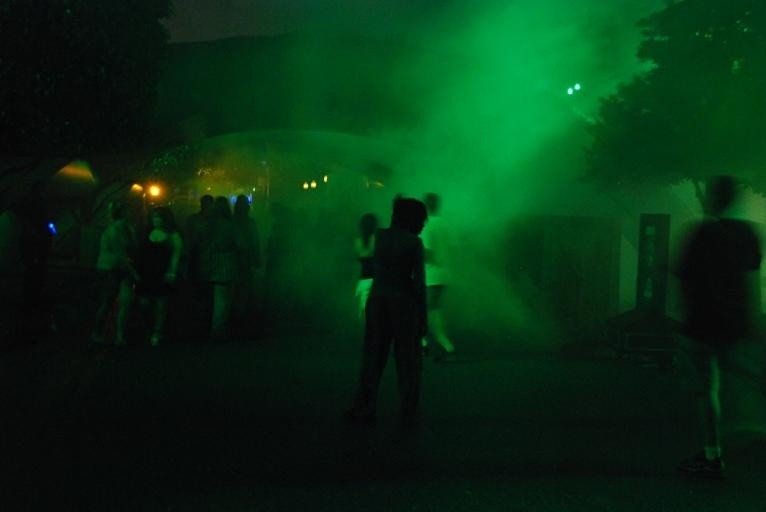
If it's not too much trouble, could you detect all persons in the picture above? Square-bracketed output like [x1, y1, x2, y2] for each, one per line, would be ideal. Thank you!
[668, 175, 764, 475]
[353, 194, 453, 469]
[96, 188, 262, 350]
